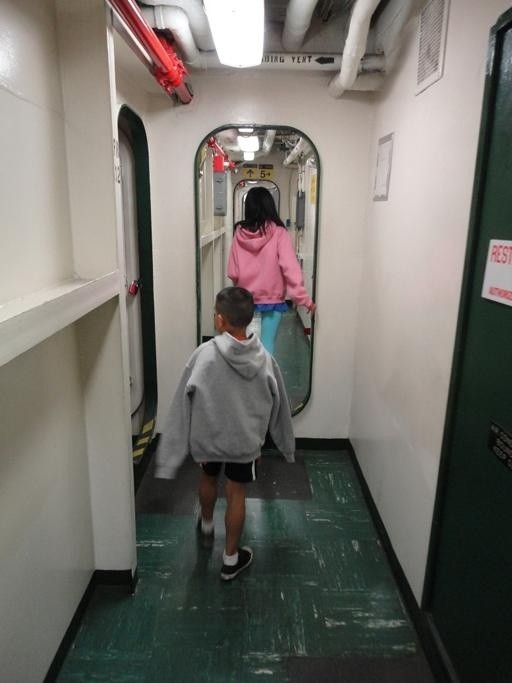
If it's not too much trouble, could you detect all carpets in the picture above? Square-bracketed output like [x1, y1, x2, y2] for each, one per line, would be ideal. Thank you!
[284, 647, 438, 682]
[136, 443, 313, 500]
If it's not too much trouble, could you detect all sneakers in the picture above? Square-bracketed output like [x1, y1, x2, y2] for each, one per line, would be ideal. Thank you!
[219, 545, 253, 580]
[197, 515, 216, 549]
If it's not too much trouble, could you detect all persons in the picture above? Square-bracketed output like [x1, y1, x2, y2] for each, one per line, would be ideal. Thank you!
[152, 286, 295, 581]
[226, 185, 315, 359]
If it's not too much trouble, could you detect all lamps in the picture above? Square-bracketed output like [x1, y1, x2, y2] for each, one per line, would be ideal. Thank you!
[236, 131, 261, 152]
[199, 0, 269, 70]
